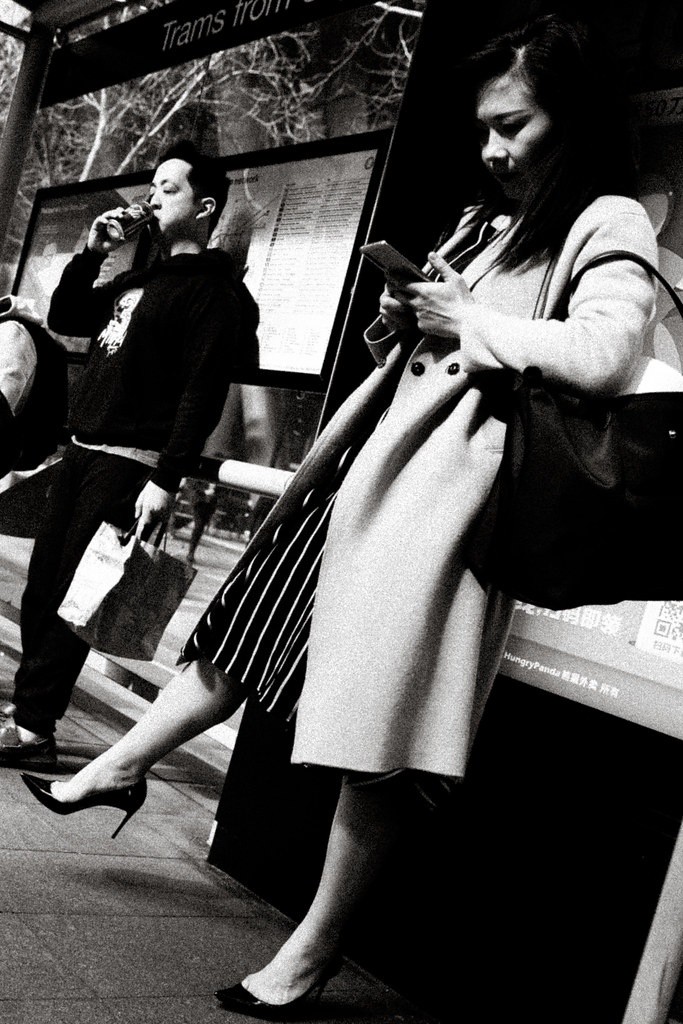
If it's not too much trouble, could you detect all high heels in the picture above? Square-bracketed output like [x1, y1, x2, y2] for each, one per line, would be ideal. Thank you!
[20, 771, 147, 839]
[215, 947, 342, 1019]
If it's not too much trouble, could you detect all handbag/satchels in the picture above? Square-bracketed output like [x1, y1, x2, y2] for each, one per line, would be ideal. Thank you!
[56, 513, 197, 662]
[463, 252, 681, 610]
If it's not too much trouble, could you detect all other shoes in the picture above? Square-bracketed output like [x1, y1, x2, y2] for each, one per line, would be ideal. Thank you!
[0, 701, 57, 762]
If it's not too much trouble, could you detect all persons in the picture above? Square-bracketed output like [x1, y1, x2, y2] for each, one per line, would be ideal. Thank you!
[0, 295, 68, 537]
[17, 33, 660, 1024]
[1, 148, 259, 769]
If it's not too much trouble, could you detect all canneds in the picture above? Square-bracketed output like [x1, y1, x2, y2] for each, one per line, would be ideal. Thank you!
[107, 201, 154, 241]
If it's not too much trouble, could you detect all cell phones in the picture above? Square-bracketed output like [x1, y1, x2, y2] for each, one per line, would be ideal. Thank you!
[360, 241, 438, 286]
